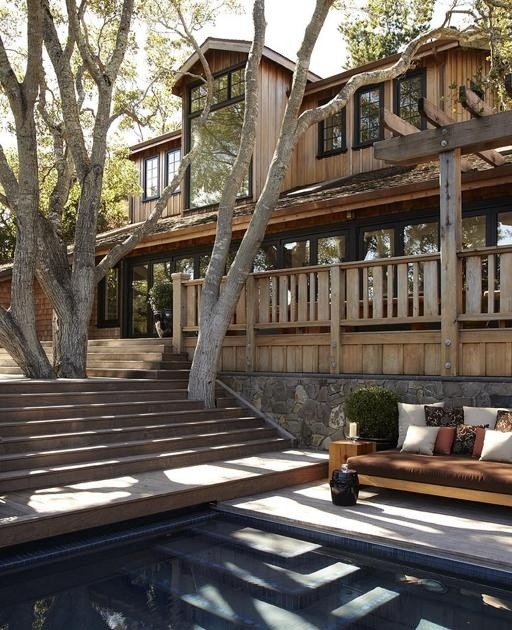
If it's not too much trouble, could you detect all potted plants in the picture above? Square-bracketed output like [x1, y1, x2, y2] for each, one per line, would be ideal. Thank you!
[148, 284, 173, 337]
[343, 387, 402, 451]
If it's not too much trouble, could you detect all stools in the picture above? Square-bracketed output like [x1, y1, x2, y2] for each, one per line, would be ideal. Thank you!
[330, 468, 359, 506]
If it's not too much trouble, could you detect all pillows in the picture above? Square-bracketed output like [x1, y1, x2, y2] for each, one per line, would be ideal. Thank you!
[397, 402, 512, 463]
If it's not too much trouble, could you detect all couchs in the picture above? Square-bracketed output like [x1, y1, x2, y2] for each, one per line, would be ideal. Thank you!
[347, 449, 512, 506]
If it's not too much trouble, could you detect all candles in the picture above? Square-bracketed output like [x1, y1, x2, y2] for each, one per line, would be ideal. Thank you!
[349, 421, 357, 437]
